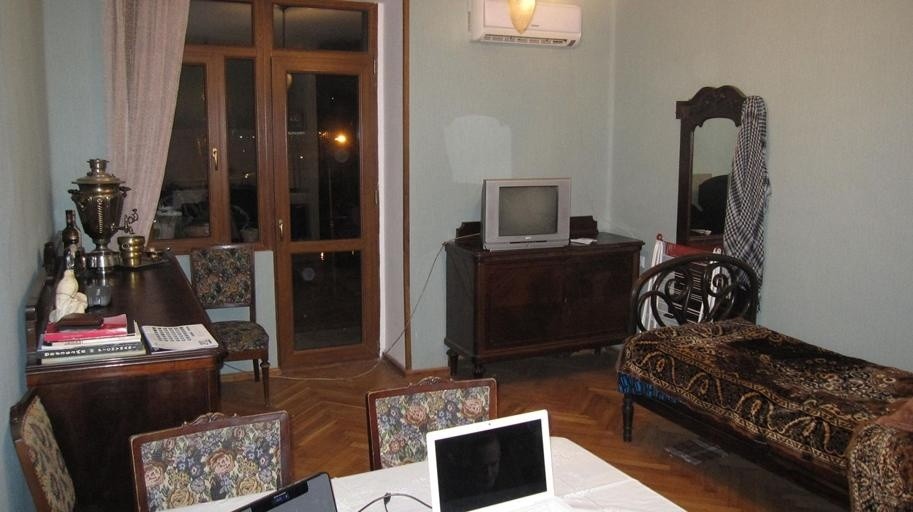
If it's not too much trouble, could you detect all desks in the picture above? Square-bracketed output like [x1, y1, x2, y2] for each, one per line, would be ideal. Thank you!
[129, 435, 686, 512]
[23, 228, 229, 512]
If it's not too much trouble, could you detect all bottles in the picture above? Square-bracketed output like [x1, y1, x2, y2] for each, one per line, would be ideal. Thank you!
[43, 210, 82, 276]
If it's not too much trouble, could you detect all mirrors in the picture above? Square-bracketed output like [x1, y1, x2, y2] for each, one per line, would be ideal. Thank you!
[661, 86, 765, 328]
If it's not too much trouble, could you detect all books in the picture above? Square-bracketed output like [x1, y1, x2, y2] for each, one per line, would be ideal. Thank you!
[37, 313, 146, 365]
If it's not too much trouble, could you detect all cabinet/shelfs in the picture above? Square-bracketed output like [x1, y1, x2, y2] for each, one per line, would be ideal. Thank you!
[445, 245, 634, 357]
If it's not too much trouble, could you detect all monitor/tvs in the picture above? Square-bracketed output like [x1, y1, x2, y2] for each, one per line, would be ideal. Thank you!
[481, 179, 571, 253]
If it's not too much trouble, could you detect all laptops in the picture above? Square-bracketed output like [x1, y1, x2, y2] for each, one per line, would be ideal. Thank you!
[425, 409, 576, 511]
[234, 471, 340, 511]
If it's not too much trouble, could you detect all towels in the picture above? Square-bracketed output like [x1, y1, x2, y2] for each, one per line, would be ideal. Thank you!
[696, 243, 726, 325]
[639, 239, 667, 336]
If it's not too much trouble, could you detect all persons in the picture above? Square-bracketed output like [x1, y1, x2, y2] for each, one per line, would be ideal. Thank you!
[438, 432, 544, 502]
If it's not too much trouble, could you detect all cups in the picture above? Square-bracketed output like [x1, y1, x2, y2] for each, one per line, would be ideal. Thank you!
[117, 236, 144, 268]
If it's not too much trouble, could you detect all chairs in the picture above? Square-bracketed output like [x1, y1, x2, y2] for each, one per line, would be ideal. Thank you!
[364, 372, 498, 473]
[9, 383, 100, 510]
[188, 245, 271, 408]
[126, 408, 293, 510]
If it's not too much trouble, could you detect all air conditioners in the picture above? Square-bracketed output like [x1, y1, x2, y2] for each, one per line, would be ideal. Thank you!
[466, 1, 582, 49]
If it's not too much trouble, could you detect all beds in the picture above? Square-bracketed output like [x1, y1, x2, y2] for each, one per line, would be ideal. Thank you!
[617, 254, 912, 512]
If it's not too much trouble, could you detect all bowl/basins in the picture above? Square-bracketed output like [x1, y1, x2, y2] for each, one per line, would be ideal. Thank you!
[142, 246, 171, 261]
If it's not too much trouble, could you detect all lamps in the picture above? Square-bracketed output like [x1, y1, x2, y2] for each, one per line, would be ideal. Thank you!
[506, 0, 537, 34]
